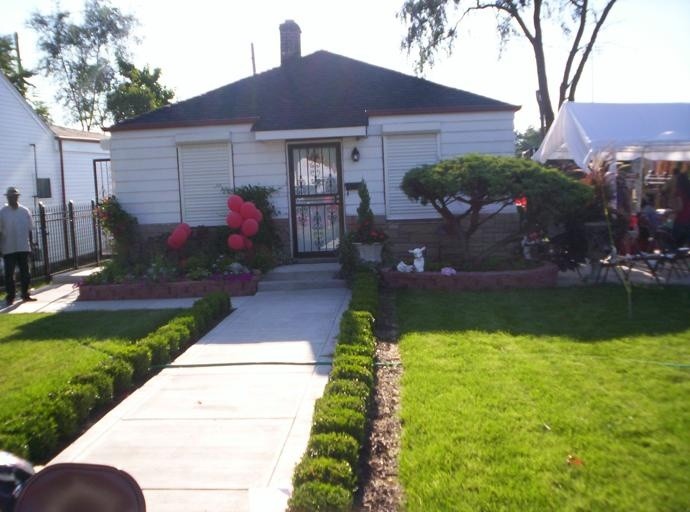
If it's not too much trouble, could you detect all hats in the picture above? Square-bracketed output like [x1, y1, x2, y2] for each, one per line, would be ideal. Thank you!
[3, 187, 20, 196]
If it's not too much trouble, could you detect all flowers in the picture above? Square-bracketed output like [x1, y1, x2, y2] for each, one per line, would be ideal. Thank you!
[347, 176, 390, 246]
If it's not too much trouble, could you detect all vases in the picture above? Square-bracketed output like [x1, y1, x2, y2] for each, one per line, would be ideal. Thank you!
[350, 242, 385, 273]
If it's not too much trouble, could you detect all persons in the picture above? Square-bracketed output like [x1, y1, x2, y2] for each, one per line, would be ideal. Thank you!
[583, 161, 690, 253]
[0, 187, 36, 304]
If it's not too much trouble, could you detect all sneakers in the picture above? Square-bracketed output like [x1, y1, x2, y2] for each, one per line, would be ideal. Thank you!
[7, 293, 37, 304]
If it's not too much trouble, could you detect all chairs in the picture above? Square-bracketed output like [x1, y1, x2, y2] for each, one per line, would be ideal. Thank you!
[11, 465, 146, 511]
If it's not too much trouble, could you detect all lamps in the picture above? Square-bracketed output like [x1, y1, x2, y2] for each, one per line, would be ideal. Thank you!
[351, 147, 360, 160]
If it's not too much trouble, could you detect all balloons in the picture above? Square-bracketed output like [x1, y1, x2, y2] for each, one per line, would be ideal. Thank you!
[166, 221, 193, 250]
[227, 196, 262, 252]
